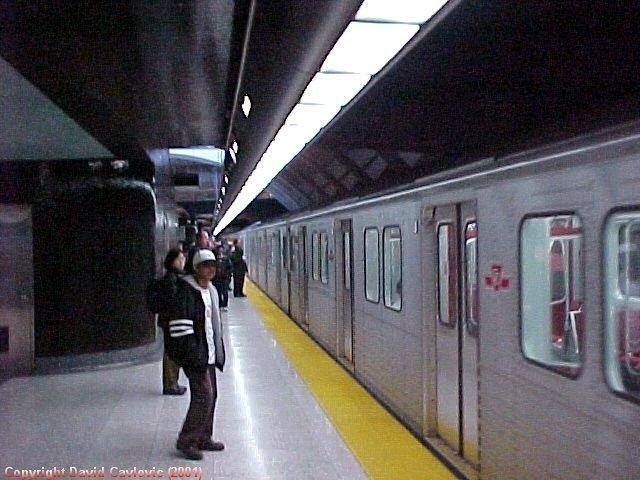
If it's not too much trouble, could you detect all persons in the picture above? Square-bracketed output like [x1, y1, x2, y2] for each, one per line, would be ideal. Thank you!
[187, 227, 247, 312]
[156, 248, 187, 394]
[168, 249, 225, 459]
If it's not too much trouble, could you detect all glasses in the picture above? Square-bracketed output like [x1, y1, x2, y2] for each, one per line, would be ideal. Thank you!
[202, 260, 215, 267]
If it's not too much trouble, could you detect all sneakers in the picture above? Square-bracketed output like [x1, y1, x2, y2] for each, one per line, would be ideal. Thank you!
[163, 385, 187, 395]
[219, 307, 228, 312]
[177, 436, 224, 459]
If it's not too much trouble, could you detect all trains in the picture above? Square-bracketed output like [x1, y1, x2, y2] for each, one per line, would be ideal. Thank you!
[220, 119, 639, 476]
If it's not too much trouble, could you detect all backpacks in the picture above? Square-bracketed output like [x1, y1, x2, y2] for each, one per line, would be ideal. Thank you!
[145, 274, 167, 314]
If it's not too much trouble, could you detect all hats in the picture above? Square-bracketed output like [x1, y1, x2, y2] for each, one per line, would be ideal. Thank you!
[193, 249, 216, 270]
